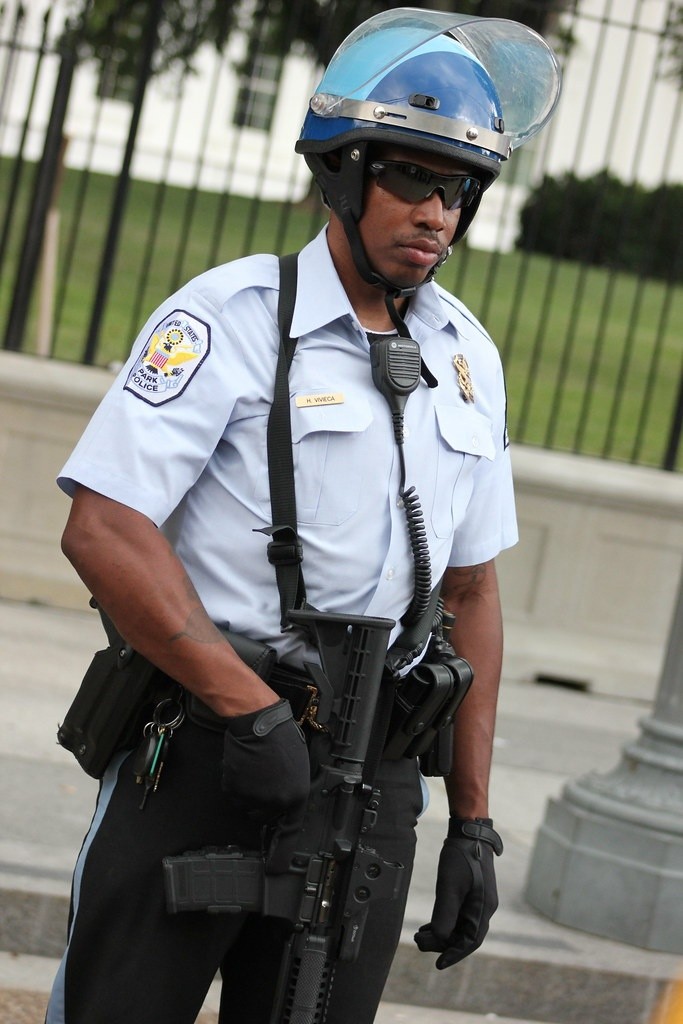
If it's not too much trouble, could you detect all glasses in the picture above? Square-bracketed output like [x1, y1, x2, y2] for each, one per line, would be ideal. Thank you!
[363, 150, 480, 210]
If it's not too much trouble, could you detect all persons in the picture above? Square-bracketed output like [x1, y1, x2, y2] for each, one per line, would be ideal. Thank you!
[43, 8, 564, 1024]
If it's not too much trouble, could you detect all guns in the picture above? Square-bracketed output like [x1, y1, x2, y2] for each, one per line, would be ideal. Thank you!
[147, 606, 400, 1024]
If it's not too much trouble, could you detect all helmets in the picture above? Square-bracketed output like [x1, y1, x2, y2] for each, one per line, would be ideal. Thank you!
[294, 6, 560, 187]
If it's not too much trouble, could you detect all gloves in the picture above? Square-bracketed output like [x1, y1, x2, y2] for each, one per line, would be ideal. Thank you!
[416, 817, 505, 969]
[215, 699, 314, 851]
